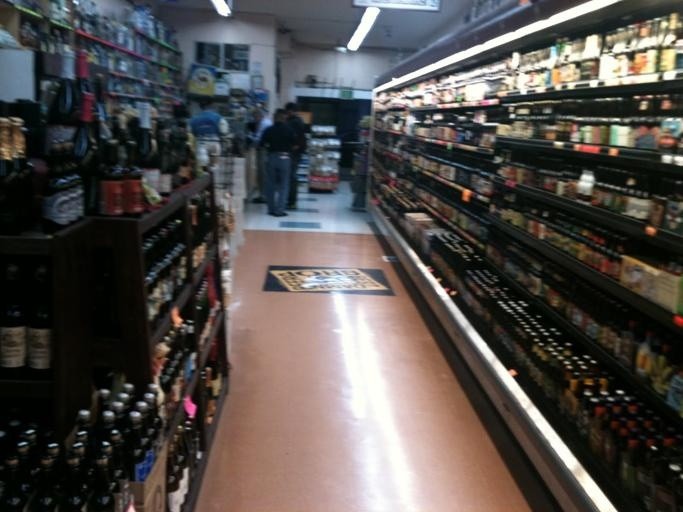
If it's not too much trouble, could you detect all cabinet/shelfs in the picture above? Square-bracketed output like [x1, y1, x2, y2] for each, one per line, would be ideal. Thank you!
[365, 0, 683, 512]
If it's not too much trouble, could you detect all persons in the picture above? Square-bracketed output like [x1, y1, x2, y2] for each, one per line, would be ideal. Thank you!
[244, 107, 274, 204]
[190, 97, 229, 136]
[337, 141, 352, 179]
[256, 108, 306, 218]
[283, 102, 305, 211]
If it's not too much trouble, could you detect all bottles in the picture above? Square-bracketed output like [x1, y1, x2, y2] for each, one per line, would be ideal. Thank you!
[0, 0, 234, 511]
[372, 9, 682, 511]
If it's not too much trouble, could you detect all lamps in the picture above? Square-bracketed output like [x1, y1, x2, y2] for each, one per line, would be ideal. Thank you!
[346, 7, 379, 52]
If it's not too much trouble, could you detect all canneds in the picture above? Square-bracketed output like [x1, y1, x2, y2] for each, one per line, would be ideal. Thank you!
[497, 114, 683, 234]
